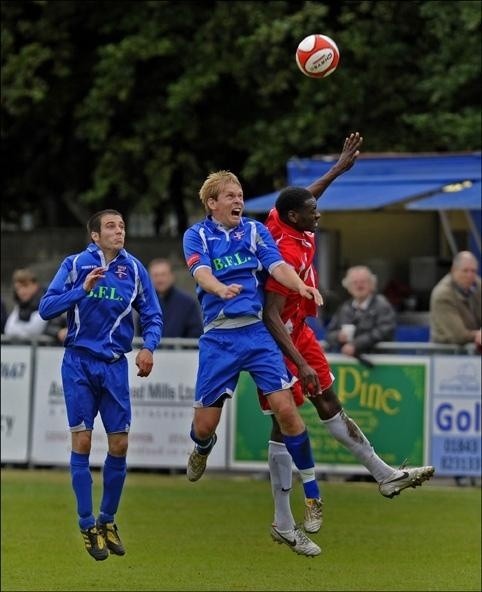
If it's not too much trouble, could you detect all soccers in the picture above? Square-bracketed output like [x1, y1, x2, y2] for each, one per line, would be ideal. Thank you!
[296, 34, 339, 78]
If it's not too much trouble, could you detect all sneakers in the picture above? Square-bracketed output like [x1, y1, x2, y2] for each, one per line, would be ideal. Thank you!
[377, 458, 436, 499]
[80, 524, 110, 561]
[187, 433, 217, 481]
[95, 517, 125, 556]
[304, 495, 324, 533]
[270, 522, 322, 558]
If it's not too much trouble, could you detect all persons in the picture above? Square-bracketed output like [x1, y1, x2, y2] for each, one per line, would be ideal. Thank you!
[324, 265, 397, 483]
[257, 132, 435, 535]
[137, 257, 204, 349]
[427, 251, 482, 487]
[183, 169, 324, 557]
[38, 208, 165, 561]
[4, 267, 49, 344]
[44, 311, 68, 346]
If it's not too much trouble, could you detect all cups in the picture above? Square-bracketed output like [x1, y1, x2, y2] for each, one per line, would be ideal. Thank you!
[341, 324, 356, 342]
